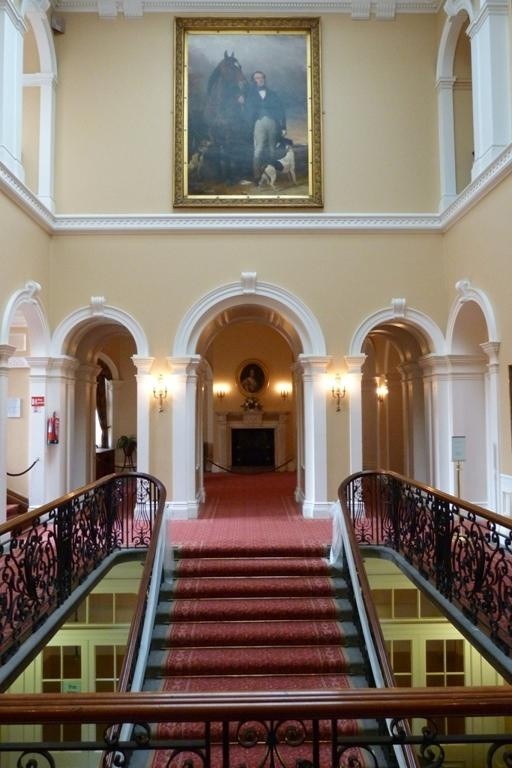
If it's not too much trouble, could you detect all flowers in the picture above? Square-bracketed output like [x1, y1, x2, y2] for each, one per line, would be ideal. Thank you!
[240, 397, 266, 414]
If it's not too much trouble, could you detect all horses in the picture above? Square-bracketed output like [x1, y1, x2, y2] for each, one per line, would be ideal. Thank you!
[205, 50, 253, 184]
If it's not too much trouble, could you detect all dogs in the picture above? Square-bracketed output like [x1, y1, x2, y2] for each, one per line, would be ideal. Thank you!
[256, 138, 298, 192]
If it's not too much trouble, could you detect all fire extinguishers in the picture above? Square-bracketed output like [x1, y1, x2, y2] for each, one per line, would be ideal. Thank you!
[47, 411, 59, 444]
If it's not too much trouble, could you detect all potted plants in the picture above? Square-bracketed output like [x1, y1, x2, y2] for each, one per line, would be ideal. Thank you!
[118, 434, 136, 457]
[241, 411, 264, 425]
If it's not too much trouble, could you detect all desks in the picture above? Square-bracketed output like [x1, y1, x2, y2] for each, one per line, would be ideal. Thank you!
[116, 463, 138, 496]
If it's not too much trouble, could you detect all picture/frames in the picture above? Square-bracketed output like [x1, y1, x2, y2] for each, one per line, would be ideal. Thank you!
[236, 358, 270, 397]
[166, 12, 327, 212]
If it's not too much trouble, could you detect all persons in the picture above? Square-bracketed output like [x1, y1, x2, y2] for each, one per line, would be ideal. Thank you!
[239, 70, 287, 185]
[242, 366, 261, 392]
